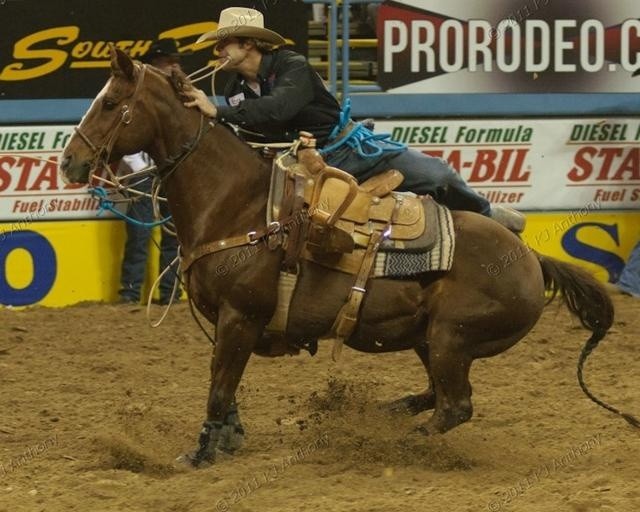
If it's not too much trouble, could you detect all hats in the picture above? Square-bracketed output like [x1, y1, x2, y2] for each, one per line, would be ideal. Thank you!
[135, 39, 193, 59]
[195, 7, 295, 46]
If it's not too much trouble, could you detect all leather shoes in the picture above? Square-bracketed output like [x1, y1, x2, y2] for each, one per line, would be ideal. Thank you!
[491, 203, 526, 232]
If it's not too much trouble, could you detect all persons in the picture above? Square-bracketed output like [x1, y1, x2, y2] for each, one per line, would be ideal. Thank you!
[616, 242, 639, 299]
[118, 38, 184, 305]
[177, 7, 527, 232]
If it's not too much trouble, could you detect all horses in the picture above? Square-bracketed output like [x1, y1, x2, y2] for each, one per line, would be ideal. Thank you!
[60, 41, 640, 471]
